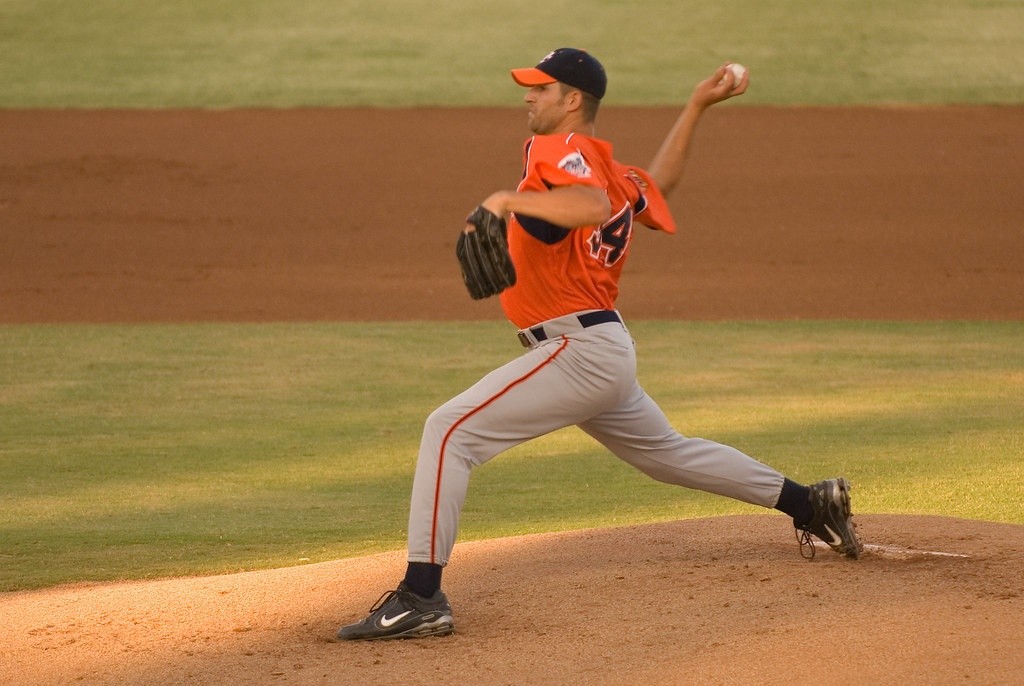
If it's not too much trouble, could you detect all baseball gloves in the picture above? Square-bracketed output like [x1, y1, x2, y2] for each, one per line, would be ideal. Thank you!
[456, 205, 517, 300]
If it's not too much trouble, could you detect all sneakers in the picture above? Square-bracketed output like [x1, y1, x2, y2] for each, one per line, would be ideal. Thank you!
[339, 580, 455, 642]
[793, 477, 863, 561]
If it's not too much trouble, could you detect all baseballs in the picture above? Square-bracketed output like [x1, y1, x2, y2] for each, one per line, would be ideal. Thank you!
[722, 64, 746, 90]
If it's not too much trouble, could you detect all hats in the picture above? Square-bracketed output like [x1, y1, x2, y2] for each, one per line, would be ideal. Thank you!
[510, 46, 608, 99]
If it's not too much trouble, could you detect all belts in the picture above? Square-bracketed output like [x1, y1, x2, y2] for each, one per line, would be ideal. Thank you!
[519, 311, 620, 348]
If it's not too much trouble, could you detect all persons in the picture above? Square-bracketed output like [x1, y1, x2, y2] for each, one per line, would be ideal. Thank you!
[334, 47, 864, 644]
